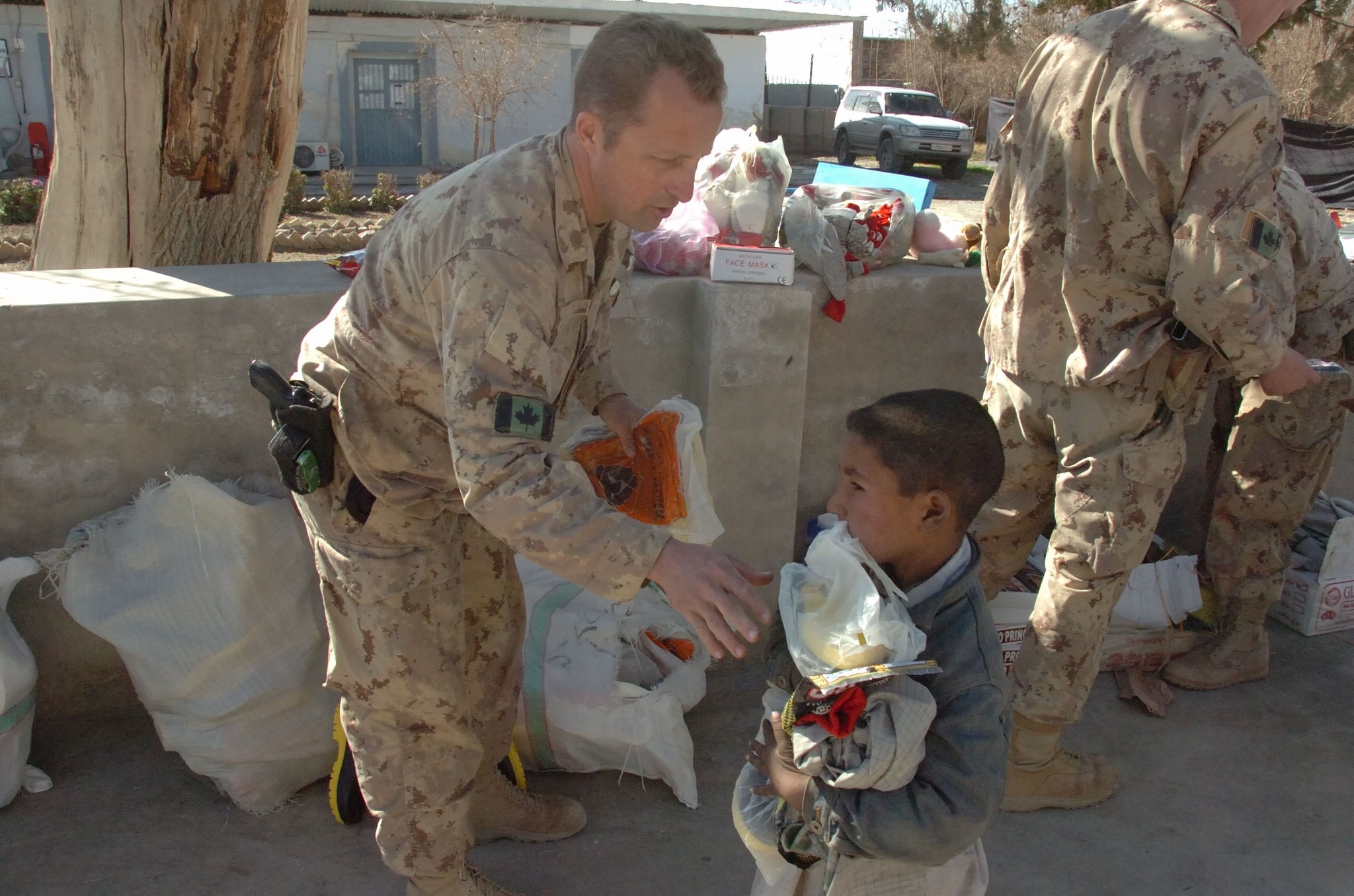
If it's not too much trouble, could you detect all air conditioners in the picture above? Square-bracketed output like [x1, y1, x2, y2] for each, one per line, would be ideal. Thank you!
[292, 141, 331, 171]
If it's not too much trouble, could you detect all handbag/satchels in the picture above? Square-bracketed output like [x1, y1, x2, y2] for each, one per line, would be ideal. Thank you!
[694, 126, 792, 247]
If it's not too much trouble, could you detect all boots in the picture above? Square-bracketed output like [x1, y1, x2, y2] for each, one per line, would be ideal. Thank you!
[998, 708, 1119, 811]
[405, 857, 517, 896]
[1161, 598, 1272, 690]
[469, 764, 587, 847]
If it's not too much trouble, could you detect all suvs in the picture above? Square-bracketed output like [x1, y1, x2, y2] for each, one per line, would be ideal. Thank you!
[831, 85, 975, 181]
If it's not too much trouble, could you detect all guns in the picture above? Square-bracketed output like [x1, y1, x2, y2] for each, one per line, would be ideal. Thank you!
[248, 358, 336, 497]
[1152, 319, 1212, 426]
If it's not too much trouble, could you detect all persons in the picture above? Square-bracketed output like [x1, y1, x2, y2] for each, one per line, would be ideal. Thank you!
[1162, 159, 1354, 690]
[272, 13, 774, 896]
[979, 0, 1321, 812]
[744, 389, 1007, 896]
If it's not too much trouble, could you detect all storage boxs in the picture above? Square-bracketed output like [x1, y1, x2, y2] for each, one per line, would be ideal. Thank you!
[965, 515, 1203, 681]
[1267, 517, 1354, 637]
[709, 241, 795, 286]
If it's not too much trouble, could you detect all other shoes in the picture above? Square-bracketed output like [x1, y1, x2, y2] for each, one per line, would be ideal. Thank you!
[497, 741, 526, 790]
[328, 700, 363, 825]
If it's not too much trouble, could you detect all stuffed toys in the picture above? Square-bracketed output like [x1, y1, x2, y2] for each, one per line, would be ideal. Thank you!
[909, 209, 983, 269]
[634, 130, 980, 300]
